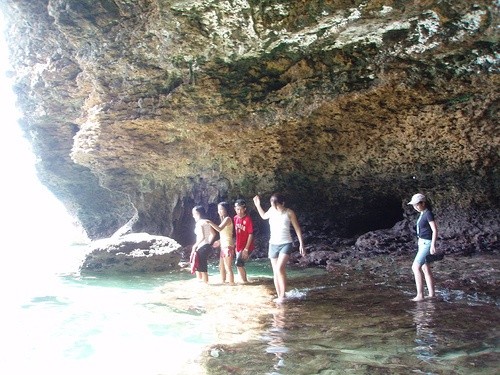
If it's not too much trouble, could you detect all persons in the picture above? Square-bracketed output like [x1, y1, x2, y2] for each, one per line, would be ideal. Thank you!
[234, 199, 255, 284]
[189, 206, 216, 284]
[253, 192, 306, 303]
[205, 201, 236, 285]
[407, 193, 438, 302]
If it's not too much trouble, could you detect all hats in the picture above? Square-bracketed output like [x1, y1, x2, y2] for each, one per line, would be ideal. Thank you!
[407, 193, 426, 205]
